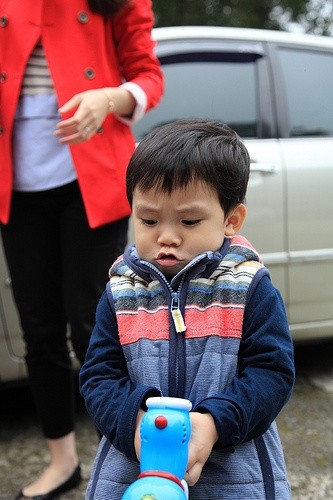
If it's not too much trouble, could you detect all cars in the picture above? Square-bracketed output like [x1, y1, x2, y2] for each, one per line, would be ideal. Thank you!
[2, 24, 332, 386]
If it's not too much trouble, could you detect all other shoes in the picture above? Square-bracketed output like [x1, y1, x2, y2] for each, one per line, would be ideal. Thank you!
[15, 460, 82, 500]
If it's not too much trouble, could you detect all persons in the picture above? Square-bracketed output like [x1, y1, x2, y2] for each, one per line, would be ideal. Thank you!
[0, 0, 166, 499]
[78, 117, 296, 500]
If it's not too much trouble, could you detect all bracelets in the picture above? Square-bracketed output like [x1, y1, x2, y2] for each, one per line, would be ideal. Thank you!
[103, 88, 114, 113]
